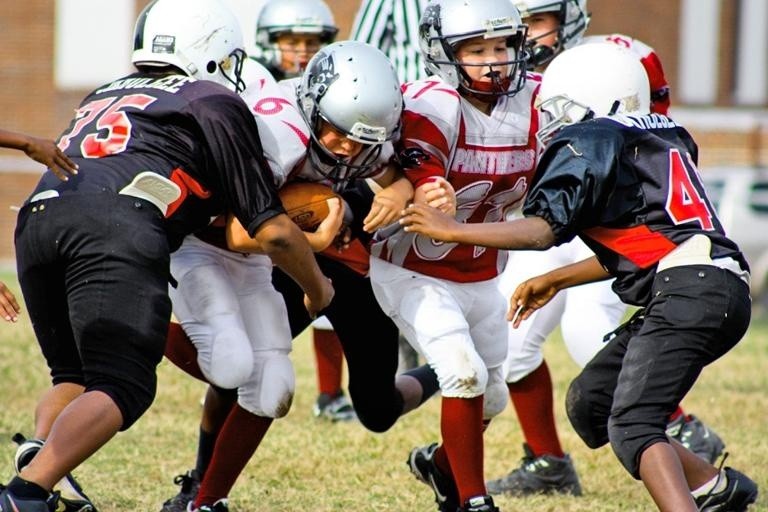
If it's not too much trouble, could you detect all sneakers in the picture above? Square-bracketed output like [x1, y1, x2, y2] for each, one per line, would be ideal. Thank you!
[407, 442, 458, 511]
[456, 494, 500, 512]
[160, 469, 202, 511]
[186, 497, 229, 512]
[690, 450, 759, 511]
[666, 415, 726, 467]
[486, 443, 582, 499]
[0, 484, 61, 512]
[12, 433, 100, 511]
[312, 389, 359, 423]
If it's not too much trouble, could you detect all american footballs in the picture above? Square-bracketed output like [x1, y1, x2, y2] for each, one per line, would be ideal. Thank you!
[278, 182, 343, 232]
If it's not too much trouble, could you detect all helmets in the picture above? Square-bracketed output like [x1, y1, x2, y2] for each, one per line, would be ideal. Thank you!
[132, 0, 248, 96]
[533, 41, 653, 152]
[255, 0, 339, 69]
[419, 0, 532, 101]
[515, 0, 592, 67]
[295, 40, 405, 183]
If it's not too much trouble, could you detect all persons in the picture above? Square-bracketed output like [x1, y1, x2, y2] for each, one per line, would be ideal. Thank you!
[0, 0, 336, 511]
[155, 1, 540, 511]
[485, 1, 726, 501]
[398, 38, 761, 512]
[0, 129, 81, 324]
